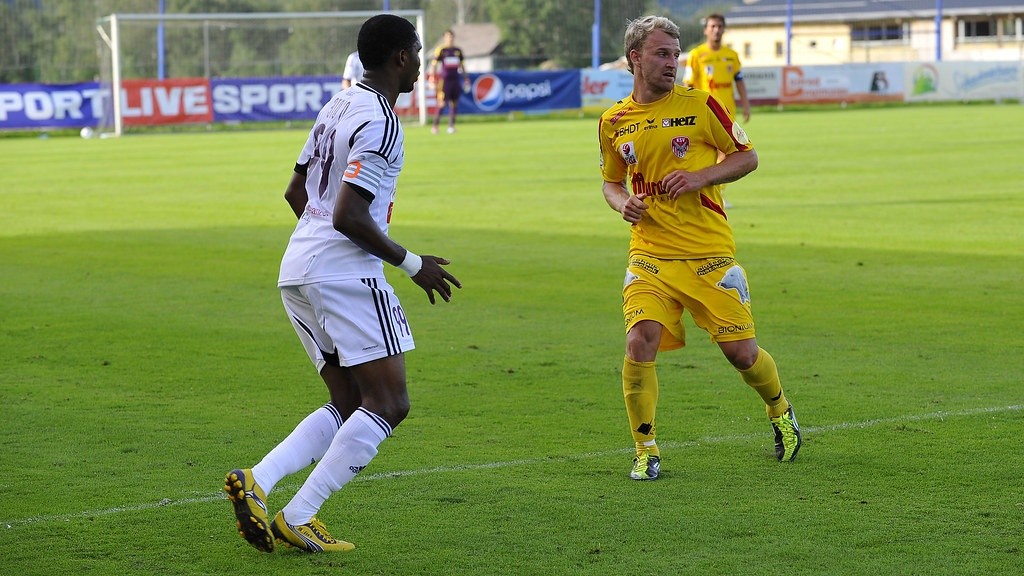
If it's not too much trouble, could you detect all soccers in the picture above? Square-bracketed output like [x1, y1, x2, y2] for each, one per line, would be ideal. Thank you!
[79, 127, 94, 139]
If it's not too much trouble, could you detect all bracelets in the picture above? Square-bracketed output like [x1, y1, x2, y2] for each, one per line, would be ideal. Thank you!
[396, 251, 423, 277]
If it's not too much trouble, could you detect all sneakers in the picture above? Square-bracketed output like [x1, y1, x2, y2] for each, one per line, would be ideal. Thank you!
[224, 469, 275, 553]
[630, 448, 660, 480]
[270, 511, 355, 552]
[767, 398, 802, 463]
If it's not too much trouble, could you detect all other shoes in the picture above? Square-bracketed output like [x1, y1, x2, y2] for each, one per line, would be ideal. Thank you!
[430, 126, 441, 134]
[447, 127, 456, 134]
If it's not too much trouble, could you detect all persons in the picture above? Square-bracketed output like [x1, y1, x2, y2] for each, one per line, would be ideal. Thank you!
[599, 16, 802, 480]
[683, 14, 750, 207]
[223, 15, 462, 554]
[343, 50, 364, 89]
[430, 30, 471, 133]
